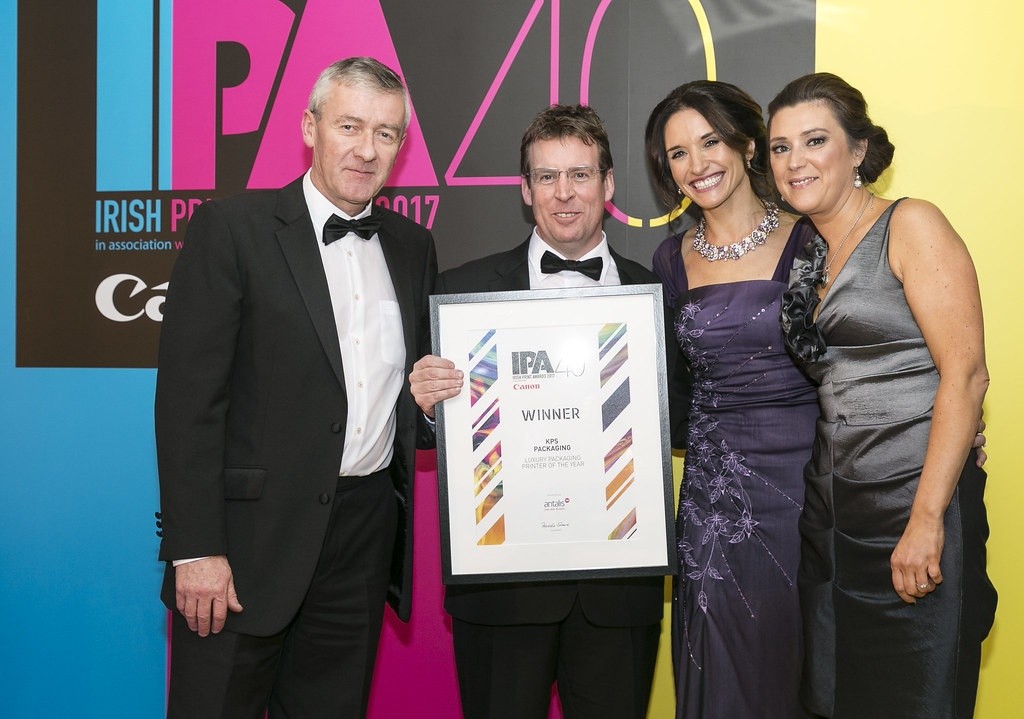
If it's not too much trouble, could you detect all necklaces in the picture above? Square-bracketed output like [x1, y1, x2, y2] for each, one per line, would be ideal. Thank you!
[693, 199, 780, 262]
[819, 193, 874, 288]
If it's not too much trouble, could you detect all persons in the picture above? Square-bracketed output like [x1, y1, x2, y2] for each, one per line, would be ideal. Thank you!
[409, 103, 665, 718]
[643, 80, 987, 719]
[765, 73, 1000, 719]
[154, 61, 440, 719]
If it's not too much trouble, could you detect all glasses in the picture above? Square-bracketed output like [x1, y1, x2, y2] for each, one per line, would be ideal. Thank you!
[525, 165, 607, 185]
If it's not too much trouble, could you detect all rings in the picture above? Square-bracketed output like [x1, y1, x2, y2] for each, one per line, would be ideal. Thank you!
[916, 582, 928, 589]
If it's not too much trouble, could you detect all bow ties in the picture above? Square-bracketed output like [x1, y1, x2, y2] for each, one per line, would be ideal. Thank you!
[540, 251, 604, 281]
[322, 212, 385, 245]
